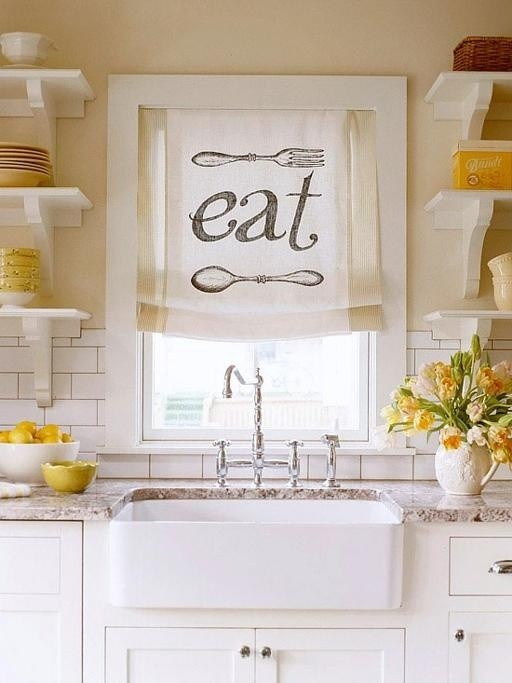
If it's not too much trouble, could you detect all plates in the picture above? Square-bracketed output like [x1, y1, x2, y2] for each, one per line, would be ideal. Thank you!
[0, 64, 50, 69]
[0, 140, 53, 188]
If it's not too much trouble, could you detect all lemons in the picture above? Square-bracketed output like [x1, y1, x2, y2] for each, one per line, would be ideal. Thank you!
[0, 421, 74, 443]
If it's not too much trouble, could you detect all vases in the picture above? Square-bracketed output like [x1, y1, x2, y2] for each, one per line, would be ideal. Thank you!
[432, 443, 500, 499]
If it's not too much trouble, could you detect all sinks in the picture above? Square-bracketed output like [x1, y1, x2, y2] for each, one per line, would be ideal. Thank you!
[108, 484, 408, 527]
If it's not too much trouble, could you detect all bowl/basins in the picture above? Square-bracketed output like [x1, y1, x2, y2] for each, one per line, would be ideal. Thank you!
[0, 441, 81, 488]
[42, 461, 96, 494]
[0, 31, 57, 64]
[0, 248, 40, 307]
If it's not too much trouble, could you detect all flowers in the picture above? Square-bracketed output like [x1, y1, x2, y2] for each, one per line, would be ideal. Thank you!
[384, 336, 511, 466]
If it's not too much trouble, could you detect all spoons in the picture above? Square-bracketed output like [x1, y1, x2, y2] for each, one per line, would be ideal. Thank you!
[189, 268, 324, 292]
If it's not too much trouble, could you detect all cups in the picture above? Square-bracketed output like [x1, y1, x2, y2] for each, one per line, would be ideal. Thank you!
[486, 254, 512, 278]
[492, 278, 512, 311]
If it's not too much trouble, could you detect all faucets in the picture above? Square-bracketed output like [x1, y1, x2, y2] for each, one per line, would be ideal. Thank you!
[221, 363, 265, 485]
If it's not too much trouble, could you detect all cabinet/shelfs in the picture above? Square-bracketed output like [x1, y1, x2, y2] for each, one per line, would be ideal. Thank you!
[1, 71, 97, 401]
[422, 71, 512, 346]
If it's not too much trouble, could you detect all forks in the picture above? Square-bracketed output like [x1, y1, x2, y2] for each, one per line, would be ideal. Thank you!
[190, 147, 325, 169]
